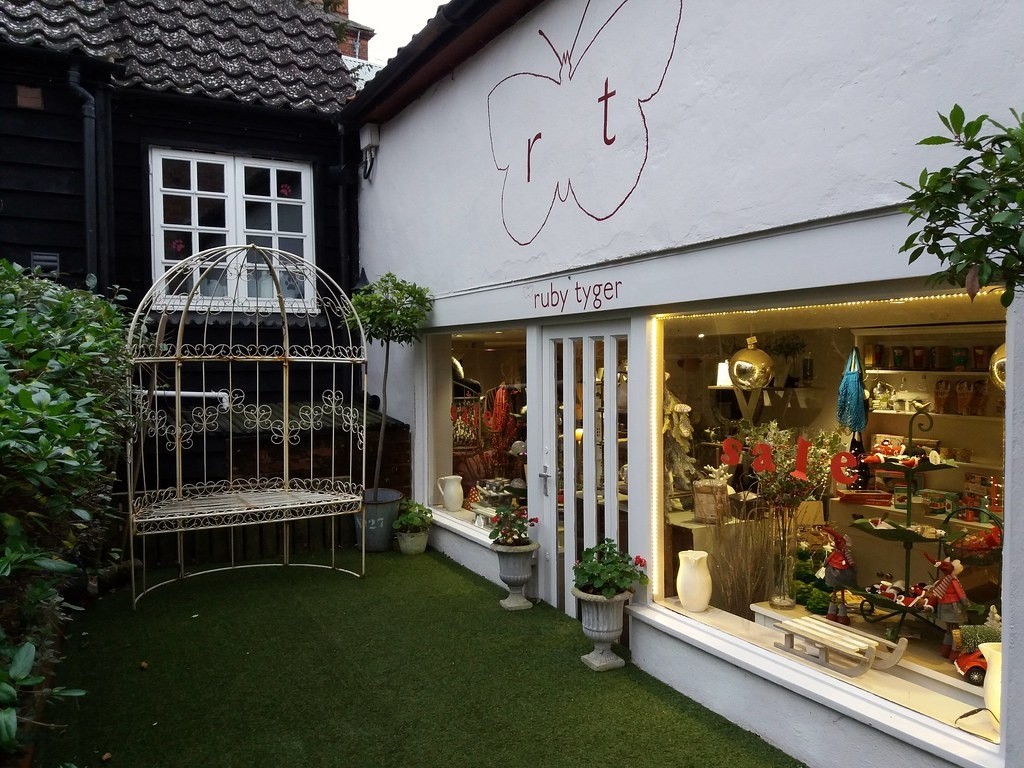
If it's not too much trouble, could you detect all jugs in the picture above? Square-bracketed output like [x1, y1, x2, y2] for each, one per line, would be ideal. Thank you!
[676, 550, 712, 611]
[437, 475, 463, 512]
[978, 642, 1003, 735]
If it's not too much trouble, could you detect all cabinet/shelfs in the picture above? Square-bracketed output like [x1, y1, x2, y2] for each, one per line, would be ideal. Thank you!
[702, 384, 825, 486]
[830, 328, 1006, 588]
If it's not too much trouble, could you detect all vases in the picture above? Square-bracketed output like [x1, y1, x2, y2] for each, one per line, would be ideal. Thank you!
[768, 506, 799, 609]
[571, 585, 632, 671]
[489, 538, 540, 610]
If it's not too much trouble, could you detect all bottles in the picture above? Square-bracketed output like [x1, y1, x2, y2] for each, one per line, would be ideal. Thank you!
[917, 375, 929, 392]
[898, 378, 909, 393]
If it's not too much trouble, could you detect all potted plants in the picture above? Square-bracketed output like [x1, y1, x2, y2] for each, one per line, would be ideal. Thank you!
[392, 499, 434, 555]
[343, 271, 436, 553]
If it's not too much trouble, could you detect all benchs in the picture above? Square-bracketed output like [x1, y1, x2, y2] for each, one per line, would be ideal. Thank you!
[133, 489, 363, 535]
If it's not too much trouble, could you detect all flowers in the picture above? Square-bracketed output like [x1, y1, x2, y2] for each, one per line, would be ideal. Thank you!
[723, 419, 841, 601]
[571, 537, 652, 599]
[488, 504, 539, 546]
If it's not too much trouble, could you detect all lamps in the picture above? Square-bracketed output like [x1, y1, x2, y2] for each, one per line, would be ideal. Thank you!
[728, 332, 775, 391]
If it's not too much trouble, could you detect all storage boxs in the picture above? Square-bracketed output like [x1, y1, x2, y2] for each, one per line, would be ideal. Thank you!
[693, 343, 1005, 525]
